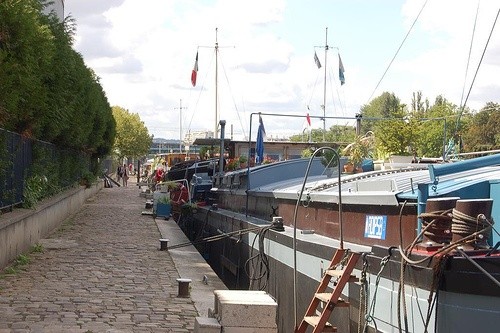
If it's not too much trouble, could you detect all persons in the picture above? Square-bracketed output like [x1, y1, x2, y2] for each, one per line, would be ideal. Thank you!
[154, 167, 165, 182]
[117, 161, 137, 187]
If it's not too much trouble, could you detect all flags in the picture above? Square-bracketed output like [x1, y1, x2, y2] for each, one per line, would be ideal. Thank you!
[254, 125, 264, 165]
[191, 51, 199, 87]
[313, 54, 322, 69]
[307, 114, 311, 126]
[337, 54, 346, 86]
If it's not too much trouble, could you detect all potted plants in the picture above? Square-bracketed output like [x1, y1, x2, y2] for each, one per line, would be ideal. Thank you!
[341, 131, 376, 174]
[157, 196, 171, 216]
[322, 148, 340, 178]
[191, 203, 198, 214]
[227, 154, 275, 171]
[82, 171, 96, 188]
